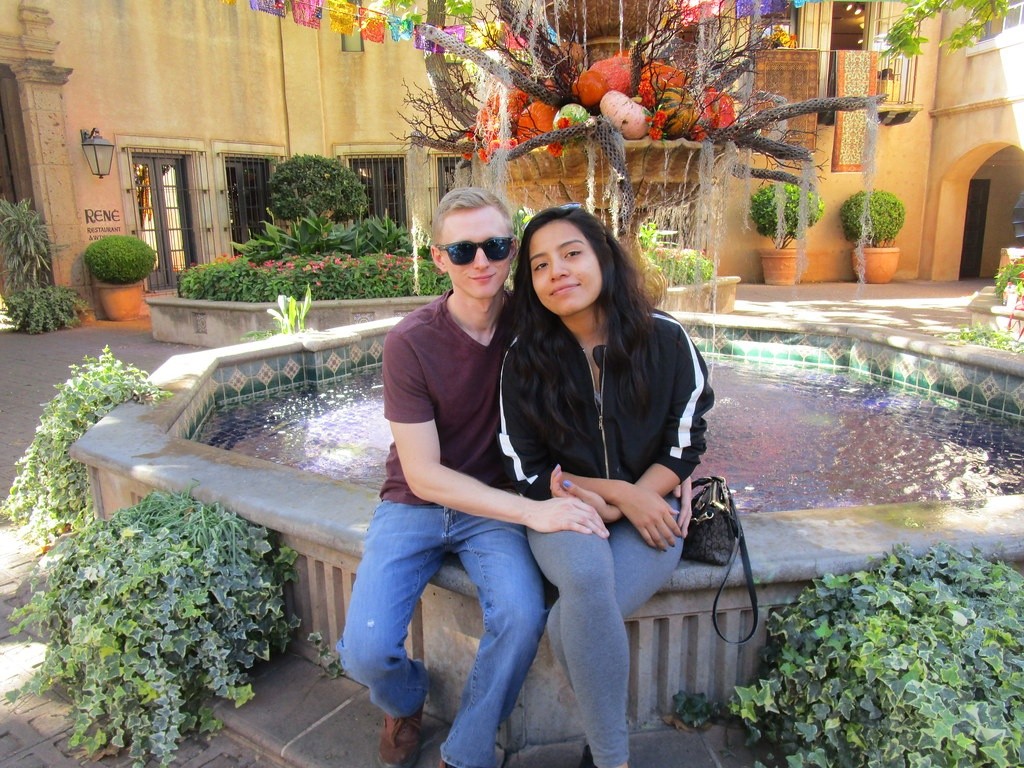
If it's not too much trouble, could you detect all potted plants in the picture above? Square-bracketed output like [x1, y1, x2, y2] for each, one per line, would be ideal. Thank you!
[749, 182, 825, 285]
[85, 235, 158, 320]
[841, 189, 907, 283]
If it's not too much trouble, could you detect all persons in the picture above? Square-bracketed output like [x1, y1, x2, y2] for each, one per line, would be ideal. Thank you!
[337, 188, 694, 768]
[496, 203, 714, 768]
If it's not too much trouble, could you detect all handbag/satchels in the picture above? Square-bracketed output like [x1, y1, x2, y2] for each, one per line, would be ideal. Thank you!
[681, 475, 738, 565]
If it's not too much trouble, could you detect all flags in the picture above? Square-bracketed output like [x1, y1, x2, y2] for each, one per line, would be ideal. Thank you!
[249, 0, 560, 54]
[680, 0, 824, 27]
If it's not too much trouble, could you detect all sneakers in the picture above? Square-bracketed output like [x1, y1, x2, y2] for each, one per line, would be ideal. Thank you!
[439, 759, 448, 768]
[377, 697, 423, 768]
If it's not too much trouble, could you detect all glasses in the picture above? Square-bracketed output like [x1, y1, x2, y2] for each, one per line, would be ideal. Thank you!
[436, 237, 515, 266]
[520, 202, 585, 232]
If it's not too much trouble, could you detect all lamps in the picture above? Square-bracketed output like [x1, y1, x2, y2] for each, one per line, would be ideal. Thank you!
[854, 2, 864, 15]
[80, 127, 116, 180]
[843, 4, 852, 11]
